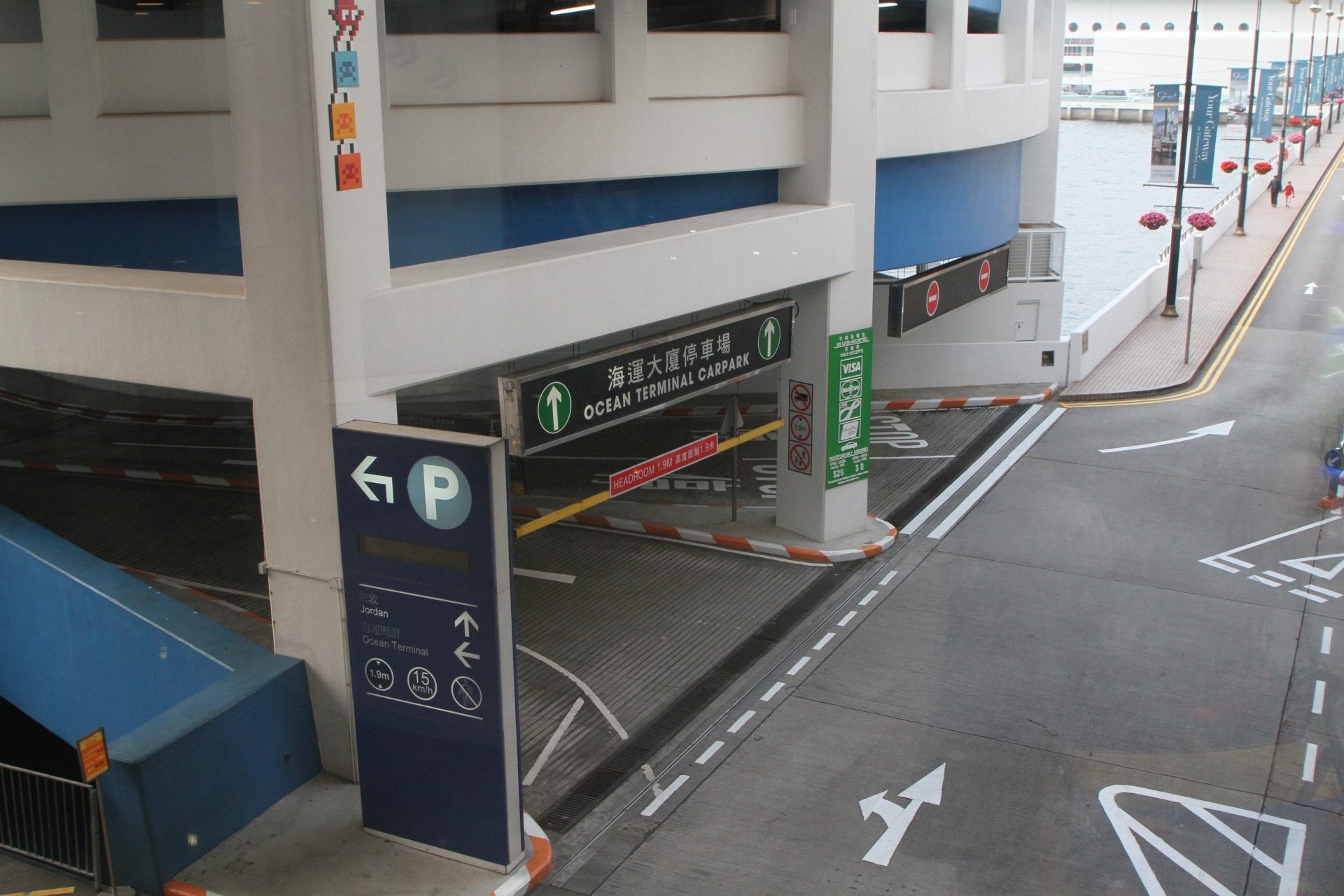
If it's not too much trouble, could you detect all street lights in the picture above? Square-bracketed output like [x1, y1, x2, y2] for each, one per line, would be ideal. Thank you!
[1299, 0, 1319, 166]
[1276, 0, 1303, 190]
[1316, 0, 1344, 147]
[1080, 62, 1086, 84]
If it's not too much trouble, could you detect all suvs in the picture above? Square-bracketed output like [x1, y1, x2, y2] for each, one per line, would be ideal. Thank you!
[1128, 88, 1145, 98]
[1092, 90, 1127, 96]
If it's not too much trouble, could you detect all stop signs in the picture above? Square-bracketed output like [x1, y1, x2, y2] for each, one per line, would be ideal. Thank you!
[926, 279, 939, 317]
[979, 260, 991, 292]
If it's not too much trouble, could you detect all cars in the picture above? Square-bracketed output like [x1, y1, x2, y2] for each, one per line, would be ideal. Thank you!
[1143, 87, 1196, 104]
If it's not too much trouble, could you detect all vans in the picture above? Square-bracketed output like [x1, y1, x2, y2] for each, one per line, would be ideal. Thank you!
[1074, 85, 1090, 95]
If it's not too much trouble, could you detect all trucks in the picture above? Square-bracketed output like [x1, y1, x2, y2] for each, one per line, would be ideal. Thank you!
[1061, 85, 1074, 93]
